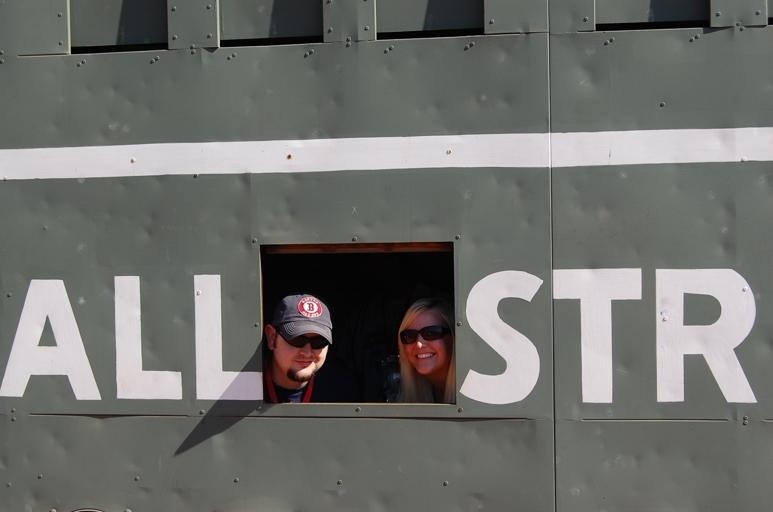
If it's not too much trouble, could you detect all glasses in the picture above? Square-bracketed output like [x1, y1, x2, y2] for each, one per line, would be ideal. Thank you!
[399, 324, 450, 345]
[279, 333, 329, 350]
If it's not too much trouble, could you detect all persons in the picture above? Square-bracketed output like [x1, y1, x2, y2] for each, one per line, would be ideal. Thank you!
[394, 296, 456, 404]
[263, 294, 333, 403]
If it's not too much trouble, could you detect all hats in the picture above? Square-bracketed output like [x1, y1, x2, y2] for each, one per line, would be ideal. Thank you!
[270, 293, 332, 344]
